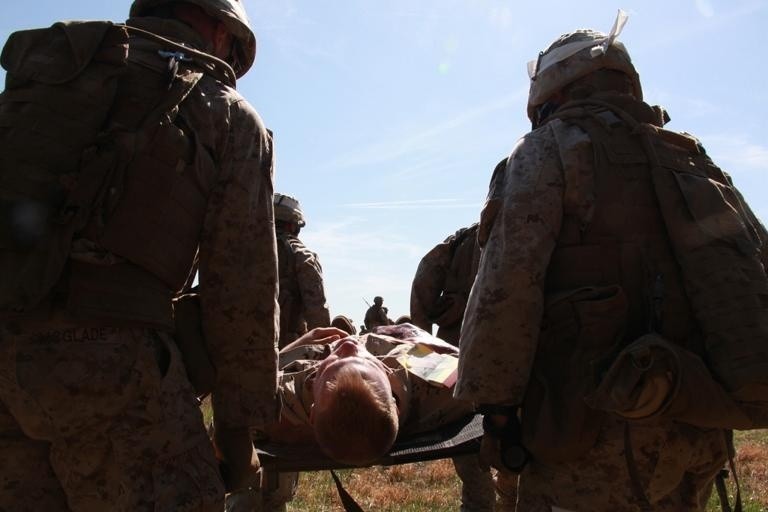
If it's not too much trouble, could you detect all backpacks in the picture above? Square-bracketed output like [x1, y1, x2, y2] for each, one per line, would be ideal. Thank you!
[547, 101, 768, 431]
[0, 19, 218, 318]
[438, 221, 481, 326]
[277, 232, 306, 330]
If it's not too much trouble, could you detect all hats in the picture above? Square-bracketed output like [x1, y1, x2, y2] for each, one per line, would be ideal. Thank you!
[270, 192, 307, 228]
[130, 0, 257, 82]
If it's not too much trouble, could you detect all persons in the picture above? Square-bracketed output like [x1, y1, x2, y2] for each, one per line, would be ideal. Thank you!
[0, 1, 282, 511]
[381, 306, 395, 325]
[217, 195, 329, 509]
[358, 326, 366, 335]
[452, 28, 768, 510]
[410, 220, 518, 511]
[364, 297, 388, 331]
[250, 324, 479, 468]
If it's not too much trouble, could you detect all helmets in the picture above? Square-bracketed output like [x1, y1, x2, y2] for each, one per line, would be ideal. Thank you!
[525, 28, 643, 119]
[373, 295, 383, 301]
